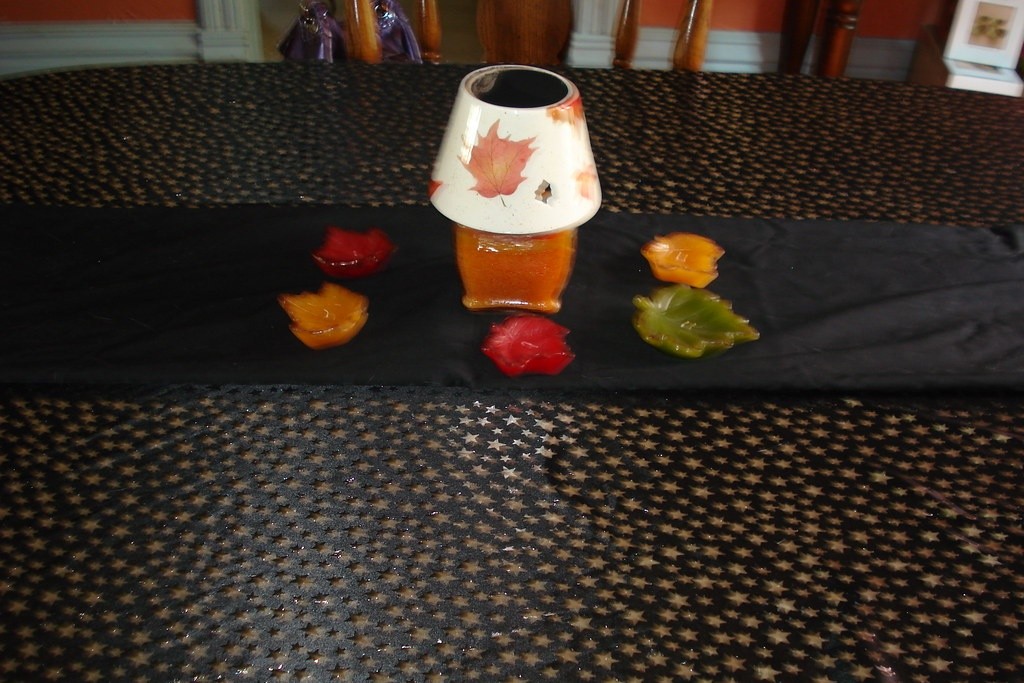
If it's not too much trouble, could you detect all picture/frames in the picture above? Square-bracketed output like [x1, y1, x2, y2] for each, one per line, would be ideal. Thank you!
[942, 0, 1024, 69]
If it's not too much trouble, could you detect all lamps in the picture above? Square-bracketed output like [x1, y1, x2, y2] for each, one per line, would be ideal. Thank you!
[426, 64, 601, 315]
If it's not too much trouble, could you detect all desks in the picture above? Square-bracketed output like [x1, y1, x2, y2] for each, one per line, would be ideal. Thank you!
[0, 53, 1024, 683]
